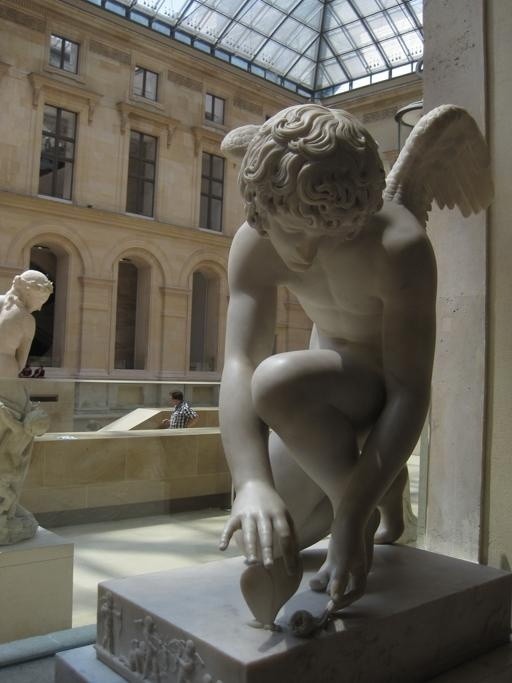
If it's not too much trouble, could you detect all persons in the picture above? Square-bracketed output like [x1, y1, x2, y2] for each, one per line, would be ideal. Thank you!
[33, 365, 45, 377]
[19, 364, 33, 379]
[0, 267, 58, 413]
[217, 99, 442, 605]
[161, 387, 200, 429]
